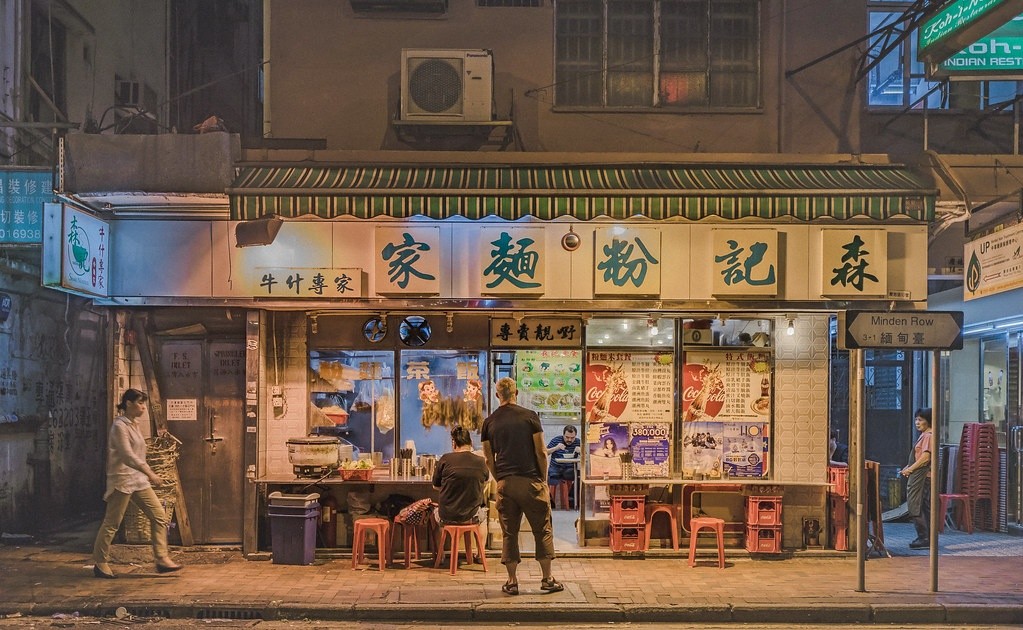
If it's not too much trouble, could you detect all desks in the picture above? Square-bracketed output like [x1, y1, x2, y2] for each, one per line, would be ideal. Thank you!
[583, 479, 836, 486]
[554, 457, 581, 511]
[255, 473, 433, 486]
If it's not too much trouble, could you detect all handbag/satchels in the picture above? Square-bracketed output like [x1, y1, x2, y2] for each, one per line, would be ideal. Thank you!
[400, 498, 438, 526]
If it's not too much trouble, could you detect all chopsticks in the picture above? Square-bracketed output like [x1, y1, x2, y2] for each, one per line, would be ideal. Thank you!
[619, 452, 633, 463]
[400, 448, 413, 459]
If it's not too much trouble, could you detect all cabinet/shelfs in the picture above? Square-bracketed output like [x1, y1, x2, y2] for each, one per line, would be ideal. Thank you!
[309, 368, 350, 431]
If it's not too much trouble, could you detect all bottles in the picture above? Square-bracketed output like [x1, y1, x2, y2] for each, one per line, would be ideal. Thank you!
[760, 373, 769, 397]
[588, 385, 617, 424]
[685, 385, 711, 422]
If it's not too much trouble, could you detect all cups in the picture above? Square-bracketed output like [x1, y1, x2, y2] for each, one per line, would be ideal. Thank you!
[425, 458, 435, 475]
[405, 439, 418, 466]
[414, 466, 423, 476]
[621, 463, 631, 480]
[389, 458, 401, 477]
[401, 459, 412, 476]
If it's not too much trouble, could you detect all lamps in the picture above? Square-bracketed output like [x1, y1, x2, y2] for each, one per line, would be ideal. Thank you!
[234, 215, 284, 248]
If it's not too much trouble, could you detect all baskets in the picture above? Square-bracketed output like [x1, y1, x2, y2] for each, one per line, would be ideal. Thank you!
[326, 414, 350, 427]
[118, 432, 182, 543]
[338, 466, 375, 481]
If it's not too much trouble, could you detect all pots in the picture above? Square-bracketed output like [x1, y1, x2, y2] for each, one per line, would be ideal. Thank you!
[286, 433, 339, 467]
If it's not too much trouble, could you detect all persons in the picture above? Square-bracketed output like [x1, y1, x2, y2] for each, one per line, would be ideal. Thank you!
[546, 425, 581, 509]
[480, 377, 564, 595]
[683, 432, 717, 450]
[92, 388, 184, 578]
[430, 425, 488, 564]
[830, 429, 849, 467]
[898, 407, 932, 550]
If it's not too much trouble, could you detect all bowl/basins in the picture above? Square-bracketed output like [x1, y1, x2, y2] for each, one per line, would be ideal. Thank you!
[562, 454, 575, 458]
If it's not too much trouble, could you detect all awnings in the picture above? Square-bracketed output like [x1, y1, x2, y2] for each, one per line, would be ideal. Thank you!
[225, 160, 943, 224]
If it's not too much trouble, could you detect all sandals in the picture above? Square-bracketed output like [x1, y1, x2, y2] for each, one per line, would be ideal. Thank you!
[540, 577, 564, 591]
[502, 581, 519, 595]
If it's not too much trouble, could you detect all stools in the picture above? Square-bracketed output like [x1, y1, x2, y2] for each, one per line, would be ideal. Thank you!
[351, 518, 488, 576]
[688, 518, 725, 570]
[643, 504, 679, 553]
[938, 422, 999, 534]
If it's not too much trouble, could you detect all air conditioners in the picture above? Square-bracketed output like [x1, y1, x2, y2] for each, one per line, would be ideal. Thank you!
[399, 48, 494, 122]
[114, 79, 158, 120]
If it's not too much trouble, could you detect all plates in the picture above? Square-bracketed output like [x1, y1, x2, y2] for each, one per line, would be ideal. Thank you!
[521, 361, 582, 409]
[750, 396, 771, 416]
[339, 445, 370, 461]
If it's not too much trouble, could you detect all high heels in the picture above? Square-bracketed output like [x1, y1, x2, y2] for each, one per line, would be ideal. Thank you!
[94, 564, 118, 579]
[157, 563, 184, 573]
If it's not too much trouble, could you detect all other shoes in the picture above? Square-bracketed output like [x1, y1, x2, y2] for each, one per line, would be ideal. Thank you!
[910, 538, 930, 550]
[433, 555, 446, 565]
[473, 554, 486, 564]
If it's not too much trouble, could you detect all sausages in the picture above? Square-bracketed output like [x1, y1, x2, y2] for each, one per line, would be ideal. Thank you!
[422, 393, 484, 434]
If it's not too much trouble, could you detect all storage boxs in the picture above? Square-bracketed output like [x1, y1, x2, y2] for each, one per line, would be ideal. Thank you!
[609, 495, 646, 553]
[827, 466, 850, 551]
[742, 496, 783, 553]
